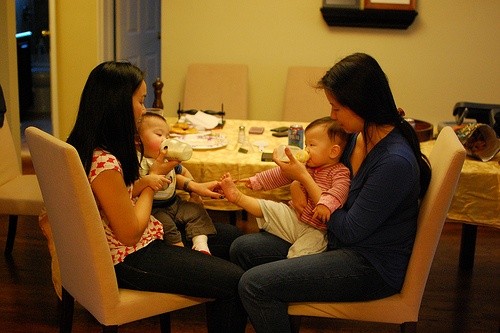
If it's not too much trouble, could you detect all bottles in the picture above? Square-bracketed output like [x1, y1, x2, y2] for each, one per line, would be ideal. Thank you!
[160, 138, 192, 161]
[277, 144, 310, 163]
[238, 125, 245, 143]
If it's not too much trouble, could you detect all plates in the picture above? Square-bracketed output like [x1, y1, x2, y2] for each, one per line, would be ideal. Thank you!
[174, 134, 228, 150]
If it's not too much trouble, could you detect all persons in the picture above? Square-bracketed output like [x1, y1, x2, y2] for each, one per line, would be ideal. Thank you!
[218, 116, 351, 260]
[131, 112, 218, 256]
[229, 53, 432, 333]
[66, 60, 247, 333]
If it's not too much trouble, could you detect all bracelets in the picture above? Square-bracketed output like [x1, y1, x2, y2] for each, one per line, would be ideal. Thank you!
[183, 178, 195, 192]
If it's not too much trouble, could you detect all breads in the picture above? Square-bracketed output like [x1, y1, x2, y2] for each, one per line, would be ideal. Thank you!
[168, 123, 197, 134]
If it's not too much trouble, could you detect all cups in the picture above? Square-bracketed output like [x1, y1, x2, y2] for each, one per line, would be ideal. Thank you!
[145, 107, 163, 116]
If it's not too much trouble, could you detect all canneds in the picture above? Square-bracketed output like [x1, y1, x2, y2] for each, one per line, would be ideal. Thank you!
[287, 124, 304, 149]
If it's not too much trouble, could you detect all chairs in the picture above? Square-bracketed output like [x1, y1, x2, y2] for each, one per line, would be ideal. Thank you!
[288, 126, 465, 333]
[182, 64, 250, 120]
[284, 66, 331, 123]
[25, 126, 213, 333]
[0, 114, 44, 256]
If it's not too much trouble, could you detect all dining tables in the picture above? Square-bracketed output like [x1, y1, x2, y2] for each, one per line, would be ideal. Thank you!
[168, 118, 500, 272]
